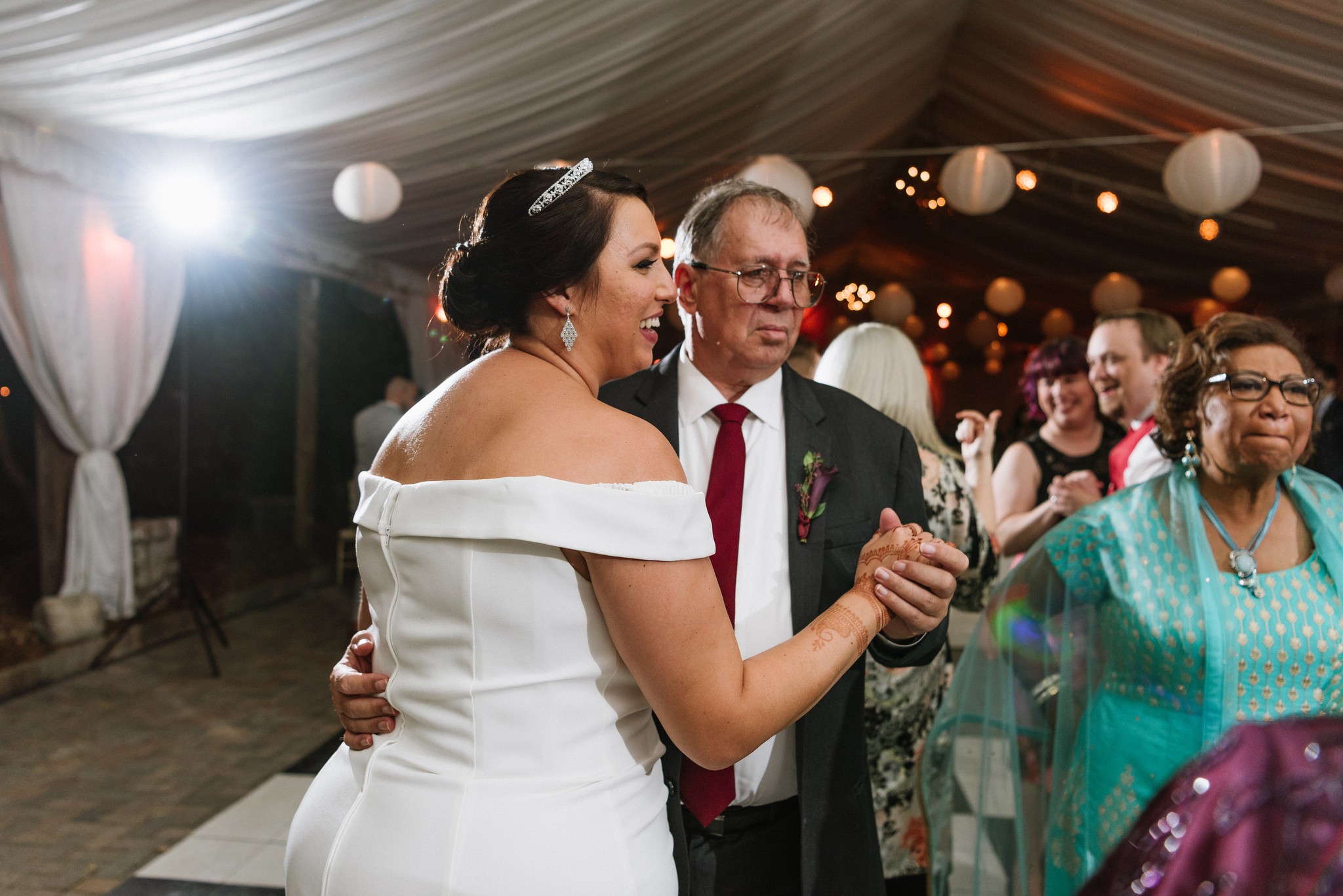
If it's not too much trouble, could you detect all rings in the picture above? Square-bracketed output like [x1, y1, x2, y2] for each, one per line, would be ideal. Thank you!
[1051, 494, 1057, 503]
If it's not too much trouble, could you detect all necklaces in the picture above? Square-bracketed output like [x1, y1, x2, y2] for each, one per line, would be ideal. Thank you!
[1197, 480, 1281, 598]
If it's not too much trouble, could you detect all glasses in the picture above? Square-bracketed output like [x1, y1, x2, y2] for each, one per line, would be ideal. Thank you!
[1198, 370, 1324, 407]
[689, 262, 826, 309]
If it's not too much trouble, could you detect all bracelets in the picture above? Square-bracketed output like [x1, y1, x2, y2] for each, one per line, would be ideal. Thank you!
[1029, 671, 1069, 706]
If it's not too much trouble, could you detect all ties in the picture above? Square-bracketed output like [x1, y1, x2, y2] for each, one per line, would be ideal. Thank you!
[1105, 414, 1158, 496]
[677, 404, 750, 829]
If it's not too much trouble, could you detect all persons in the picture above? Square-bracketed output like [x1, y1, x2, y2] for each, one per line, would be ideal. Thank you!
[787, 334, 818, 381]
[988, 337, 1128, 896]
[330, 177, 969, 895]
[809, 322, 1002, 896]
[1301, 363, 1342, 484]
[988, 311, 1342, 895]
[348, 378, 417, 514]
[1047, 309, 1184, 518]
[284, 157, 954, 896]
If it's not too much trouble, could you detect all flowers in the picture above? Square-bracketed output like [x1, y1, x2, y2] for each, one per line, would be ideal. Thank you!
[793, 450, 840, 545]
